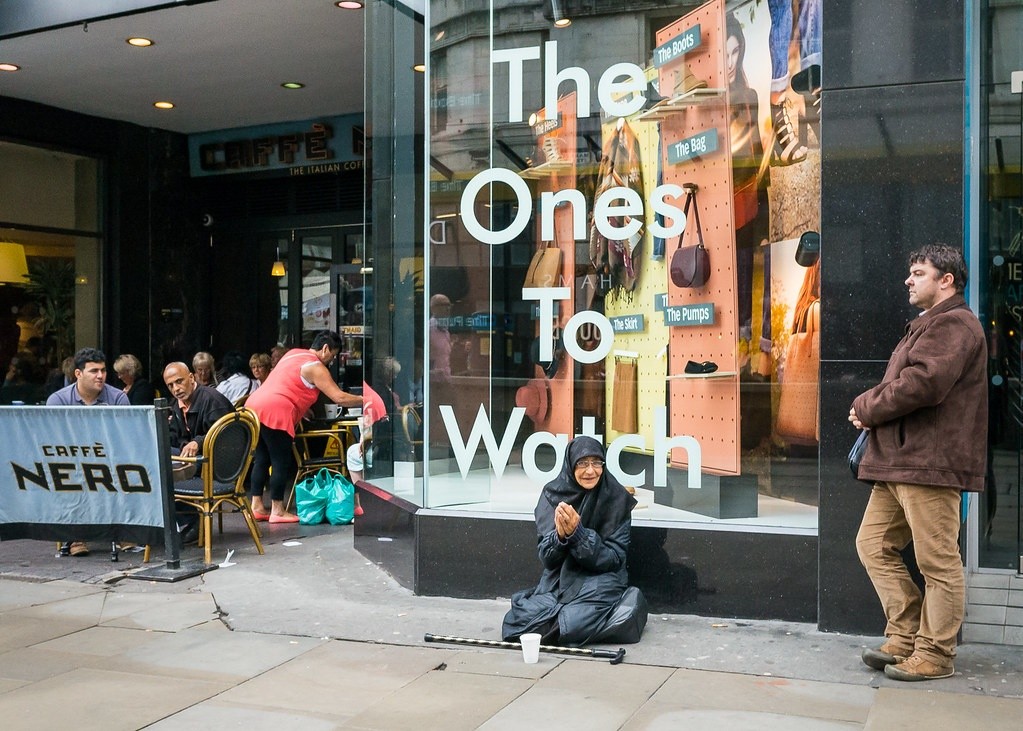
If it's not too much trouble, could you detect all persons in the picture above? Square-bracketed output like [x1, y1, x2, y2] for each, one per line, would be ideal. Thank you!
[192, 347, 289, 407]
[789, 259, 820, 333]
[150, 361, 172, 397]
[430, 293, 474, 375]
[364, 354, 402, 518]
[767, 0, 820, 167]
[501, 434, 638, 648]
[727, 17, 773, 383]
[4, 336, 77, 393]
[46, 348, 137, 555]
[245, 329, 363, 524]
[113, 353, 155, 405]
[347, 430, 372, 482]
[847, 246, 989, 680]
[163, 362, 238, 543]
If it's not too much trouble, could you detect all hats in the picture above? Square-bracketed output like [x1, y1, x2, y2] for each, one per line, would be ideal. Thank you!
[515, 378, 548, 426]
[530, 330, 563, 379]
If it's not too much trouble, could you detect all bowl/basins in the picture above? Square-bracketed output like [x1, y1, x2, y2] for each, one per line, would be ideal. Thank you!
[346, 359, 362, 366]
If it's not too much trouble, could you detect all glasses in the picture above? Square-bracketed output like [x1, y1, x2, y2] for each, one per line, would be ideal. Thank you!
[576, 460, 606, 468]
[433, 300, 454, 311]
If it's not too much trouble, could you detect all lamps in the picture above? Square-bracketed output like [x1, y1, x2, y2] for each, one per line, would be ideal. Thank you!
[270, 245, 285, 276]
[551, 0, 572, 29]
[0, 242, 31, 286]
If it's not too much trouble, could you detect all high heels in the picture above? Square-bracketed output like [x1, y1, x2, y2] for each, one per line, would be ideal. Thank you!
[797, 91, 822, 147]
[765, 102, 809, 165]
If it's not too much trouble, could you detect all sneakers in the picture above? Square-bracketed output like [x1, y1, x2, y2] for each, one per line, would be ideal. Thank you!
[884, 655, 954, 680]
[862, 647, 915, 670]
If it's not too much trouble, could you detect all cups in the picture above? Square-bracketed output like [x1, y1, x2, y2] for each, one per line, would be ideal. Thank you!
[324, 404, 342, 419]
[520, 633, 541, 663]
[11, 400, 25, 405]
[348, 408, 361, 416]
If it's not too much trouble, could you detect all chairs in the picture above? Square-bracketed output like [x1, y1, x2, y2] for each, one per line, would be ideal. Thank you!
[285, 417, 354, 515]
[143, 406, 264, 566]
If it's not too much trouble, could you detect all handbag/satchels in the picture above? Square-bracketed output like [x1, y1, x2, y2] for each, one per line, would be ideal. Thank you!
[845, 428, 876, 486]
[777, 300, 820, 446]
[294, 468, 358, 524]
[522, 227, 563, 295]
[669, 191, 711, 286]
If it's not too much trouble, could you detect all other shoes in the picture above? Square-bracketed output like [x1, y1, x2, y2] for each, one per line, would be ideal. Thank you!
[119, 541, 135, 551]
[354, 506, 364, 516]
[684, 361, 718, 374]
[672, 65, 707, 98]
[636, 84, 670, 113]
[253, 511, 270, 521]
[540, 133, 565, 164]
[180, 523, 201, 544]
[70, 541, 88, 556]
[269, 512, 299, 524]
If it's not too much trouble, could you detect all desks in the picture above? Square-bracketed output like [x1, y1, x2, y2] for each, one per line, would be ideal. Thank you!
[333, 415, 363, 455]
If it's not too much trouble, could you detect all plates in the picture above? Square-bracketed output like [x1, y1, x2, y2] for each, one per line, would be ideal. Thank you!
[349, 387, 362, 389]
[344, 415, 363, 418]
